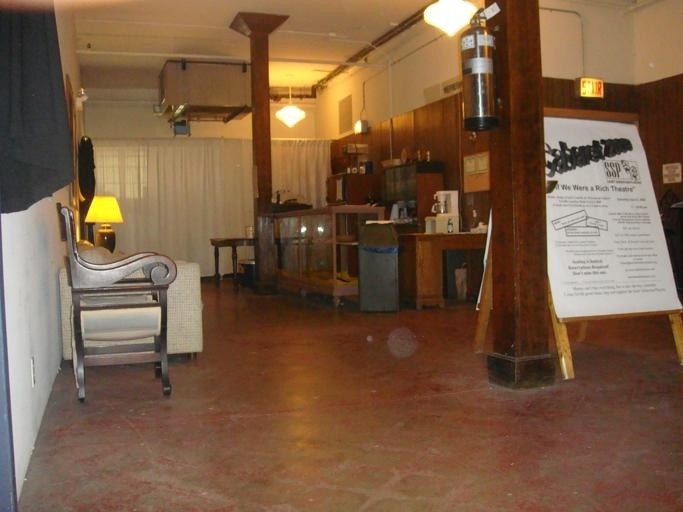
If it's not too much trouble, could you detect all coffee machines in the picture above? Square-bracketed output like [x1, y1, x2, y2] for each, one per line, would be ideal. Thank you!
[431, 189, 460, 217]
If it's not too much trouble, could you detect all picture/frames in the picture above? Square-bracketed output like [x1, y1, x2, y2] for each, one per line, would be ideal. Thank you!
[65, 73, 81, 213]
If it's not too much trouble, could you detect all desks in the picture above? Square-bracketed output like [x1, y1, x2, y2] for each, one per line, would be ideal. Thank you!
[396, 231, 488, 313]
[208, 234, 315, 296]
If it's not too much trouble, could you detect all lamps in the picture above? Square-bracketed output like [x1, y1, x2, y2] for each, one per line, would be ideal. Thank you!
[74, 86, 89, 104]
[353, 119, 368, 136]
[83, 196, 126, 255]
[422, 0, 479, 40]
[274, 79, 306, 130]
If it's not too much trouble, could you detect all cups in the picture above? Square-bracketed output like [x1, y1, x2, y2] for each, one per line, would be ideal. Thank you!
[415, 147, 433, 162]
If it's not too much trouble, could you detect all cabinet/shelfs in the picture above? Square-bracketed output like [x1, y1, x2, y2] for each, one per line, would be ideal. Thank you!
[324, 170, 381, 277]
[256, 202, 388, 306]
[381, 164, 446, 230]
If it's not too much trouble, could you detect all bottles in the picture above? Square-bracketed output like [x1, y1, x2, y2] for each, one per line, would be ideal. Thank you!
[447, 217, 453, 234]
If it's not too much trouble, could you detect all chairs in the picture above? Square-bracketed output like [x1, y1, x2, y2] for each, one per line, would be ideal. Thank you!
[62, 206, 177, 404]
[60, 238, 204, 368]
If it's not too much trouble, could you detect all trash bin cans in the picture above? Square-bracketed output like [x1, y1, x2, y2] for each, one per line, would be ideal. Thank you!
[357, 223, 401, 314]
